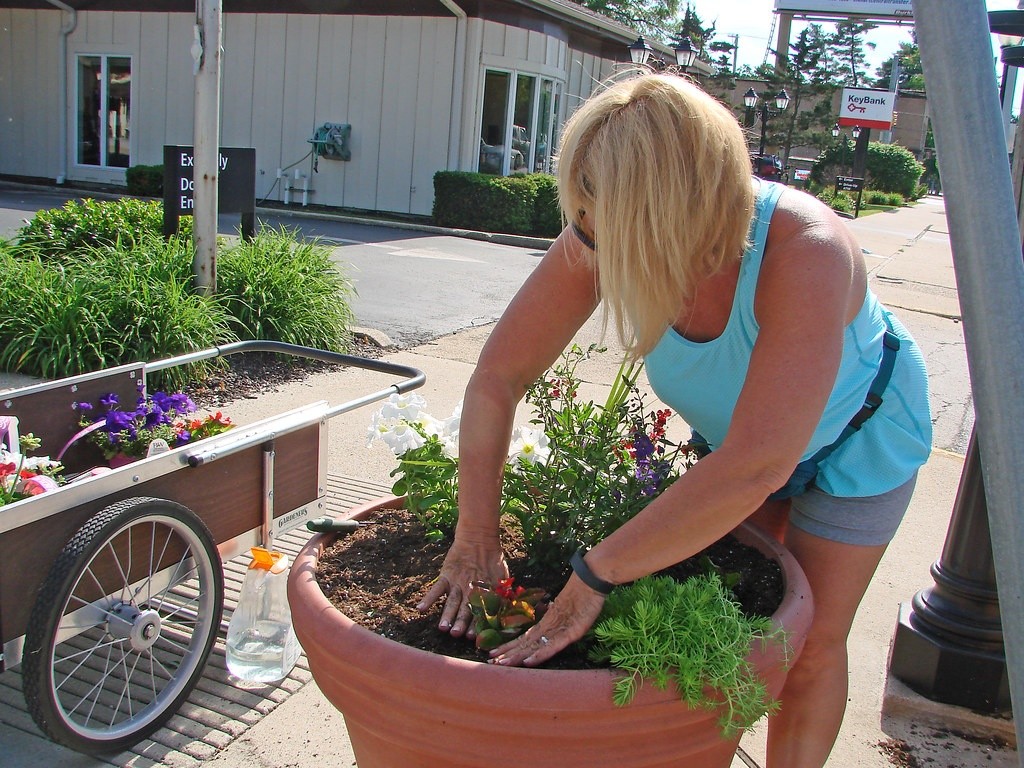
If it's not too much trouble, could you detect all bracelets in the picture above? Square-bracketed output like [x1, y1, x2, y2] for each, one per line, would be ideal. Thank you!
[569, 551, 616, 594]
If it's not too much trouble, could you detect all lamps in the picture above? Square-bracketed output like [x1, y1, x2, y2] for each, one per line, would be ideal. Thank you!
[831, 123, 840, 137]
[852, 125, 861, 139]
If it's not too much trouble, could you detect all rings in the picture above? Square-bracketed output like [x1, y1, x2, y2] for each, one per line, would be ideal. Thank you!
[541, 636, 548, 643]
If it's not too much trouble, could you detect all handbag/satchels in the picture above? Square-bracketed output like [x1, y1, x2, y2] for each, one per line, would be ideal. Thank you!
[690, 431, 818, 503]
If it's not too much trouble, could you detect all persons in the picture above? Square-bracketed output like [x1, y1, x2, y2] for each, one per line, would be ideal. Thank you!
[414, 69, 932, 768]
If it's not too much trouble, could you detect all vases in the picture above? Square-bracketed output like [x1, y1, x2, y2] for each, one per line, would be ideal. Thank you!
[287, 491, 813, 768]
[107, 446, 176, 473]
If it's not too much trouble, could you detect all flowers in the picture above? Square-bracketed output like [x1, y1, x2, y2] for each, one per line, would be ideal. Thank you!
[75, 393, 229, 458]
[0, 432, 67, 507]
[411, 360, 791, 734]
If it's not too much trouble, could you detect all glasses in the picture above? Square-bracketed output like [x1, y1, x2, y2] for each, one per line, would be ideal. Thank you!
[571, 206, 596, 251]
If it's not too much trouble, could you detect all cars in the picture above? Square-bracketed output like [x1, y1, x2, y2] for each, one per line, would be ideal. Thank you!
[480, 125, 548, 172]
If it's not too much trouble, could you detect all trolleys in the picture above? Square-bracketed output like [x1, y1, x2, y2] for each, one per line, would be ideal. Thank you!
[0, 338, 428, 758]
